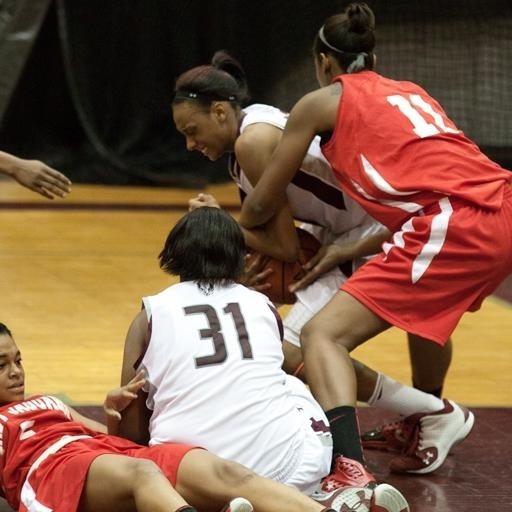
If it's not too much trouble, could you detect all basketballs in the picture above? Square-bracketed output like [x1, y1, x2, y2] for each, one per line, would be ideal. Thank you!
[247, 227, 322, 305]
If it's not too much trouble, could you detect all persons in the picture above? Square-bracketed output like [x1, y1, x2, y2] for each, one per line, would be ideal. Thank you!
[0, 149, 71, 201]
[238, 0, 511, 507]
[119, 204, 335, 498]
[172, 53, 474, 477]
[1, 320, 409, 511]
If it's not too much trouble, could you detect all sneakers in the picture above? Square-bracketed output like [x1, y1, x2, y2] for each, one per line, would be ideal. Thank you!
[221, 498, 257, 512]
[332, 482, 411, 512]
[391, 399, 475, 472]
[309, 457, 373, 502]
[361, 418, 410, 448]
